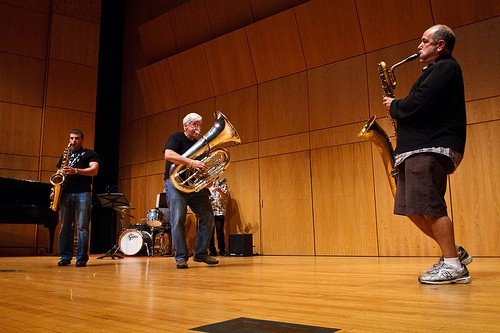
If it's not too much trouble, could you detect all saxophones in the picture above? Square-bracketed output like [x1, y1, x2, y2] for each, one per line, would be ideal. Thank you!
[50, 141, 74, 212]
[357, 50, 420, 198]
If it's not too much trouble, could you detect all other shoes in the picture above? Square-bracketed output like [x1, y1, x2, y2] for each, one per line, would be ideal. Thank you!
[219, 250, 227, 256]
[210, 252, 216, 256]
[58, 259, 71, 266]
[76, 260, 86, 267]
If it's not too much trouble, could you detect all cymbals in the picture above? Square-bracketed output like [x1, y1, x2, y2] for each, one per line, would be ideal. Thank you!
[117, 209, 136, 218]
[115, 205, 136, 209]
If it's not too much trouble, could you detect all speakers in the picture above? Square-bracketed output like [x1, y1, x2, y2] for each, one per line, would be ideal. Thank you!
[229, 233, 253, 256]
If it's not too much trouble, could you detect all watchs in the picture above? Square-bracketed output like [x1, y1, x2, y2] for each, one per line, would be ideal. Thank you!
[75, 168, 78, 175]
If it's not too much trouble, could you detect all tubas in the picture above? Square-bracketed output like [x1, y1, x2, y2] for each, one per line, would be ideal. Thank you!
[169, 110, 242, 193]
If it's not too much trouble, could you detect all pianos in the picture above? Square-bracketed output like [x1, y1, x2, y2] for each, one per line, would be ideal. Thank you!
[0, 177, 59, 256]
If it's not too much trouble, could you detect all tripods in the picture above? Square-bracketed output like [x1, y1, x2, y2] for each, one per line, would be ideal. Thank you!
[95, 192, 132, 259]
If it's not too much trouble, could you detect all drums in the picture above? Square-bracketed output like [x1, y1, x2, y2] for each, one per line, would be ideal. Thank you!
[146, 209, 164, 227]
[129, 222, 150, 240]
[119, 228, 153, 256]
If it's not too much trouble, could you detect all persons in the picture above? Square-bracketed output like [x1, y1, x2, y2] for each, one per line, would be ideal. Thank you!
[49, 129, 99, 267]
[163, 113, 219, 269]
[209, 176, 229, 256]
[383, 25, 472, 285]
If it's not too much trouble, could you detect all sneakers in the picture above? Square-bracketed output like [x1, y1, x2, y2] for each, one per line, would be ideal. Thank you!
[427, 245, 472, 273]
[418, 262, 471, 284]
[176, 259, 188, 268]
[193, 254, 219, 264]
